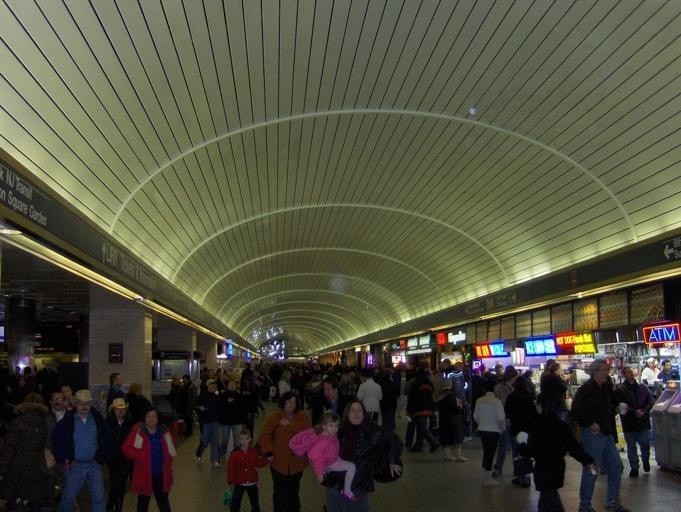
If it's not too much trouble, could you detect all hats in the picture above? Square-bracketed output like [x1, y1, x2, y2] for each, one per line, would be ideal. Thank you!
[73, 389, 93, 401]
[108, 398, 129, 414]
[206, 378, 217, 386]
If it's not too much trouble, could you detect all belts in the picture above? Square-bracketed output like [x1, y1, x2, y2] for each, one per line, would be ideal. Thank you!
[74, 459, 97, 464]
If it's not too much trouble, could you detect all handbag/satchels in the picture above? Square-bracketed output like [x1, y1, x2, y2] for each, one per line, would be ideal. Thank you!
[513, 442, 534, 476]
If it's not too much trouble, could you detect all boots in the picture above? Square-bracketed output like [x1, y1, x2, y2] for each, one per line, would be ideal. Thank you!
[442, 447, 455, 463]
[481, 467, 501, 487]
[455, 443, 469, 462]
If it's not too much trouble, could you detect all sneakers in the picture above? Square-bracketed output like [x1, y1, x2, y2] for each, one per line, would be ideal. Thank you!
[629, 467, 639, 478]
[578, 502, 597, 512]
[210, 460, 221, 468]
[340, 488, 359, 502]
[512, 476, 531, 487]
[642, 458, 651, 472]
[605, 503, 629, 511]
[405, 442, 441, 453]
[195, 453, 202, 465]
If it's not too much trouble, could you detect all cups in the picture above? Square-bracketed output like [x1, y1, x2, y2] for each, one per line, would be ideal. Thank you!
[619, 402, 629, 415]
